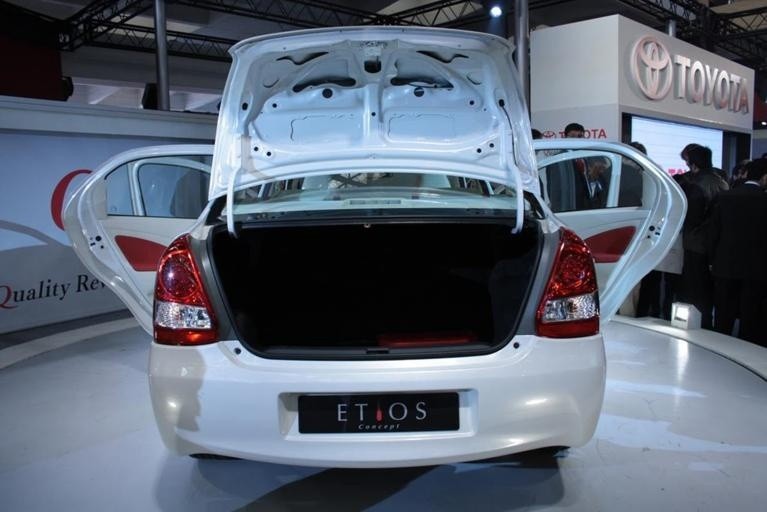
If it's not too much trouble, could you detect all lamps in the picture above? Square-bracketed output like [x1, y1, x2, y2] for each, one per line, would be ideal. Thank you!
[671, 301, 702, 329]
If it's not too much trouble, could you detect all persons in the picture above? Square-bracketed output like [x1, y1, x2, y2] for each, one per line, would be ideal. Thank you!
[532, 124, 766, 347]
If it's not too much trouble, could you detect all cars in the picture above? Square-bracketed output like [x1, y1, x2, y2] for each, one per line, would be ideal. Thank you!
[60, 23, 689, 474]
